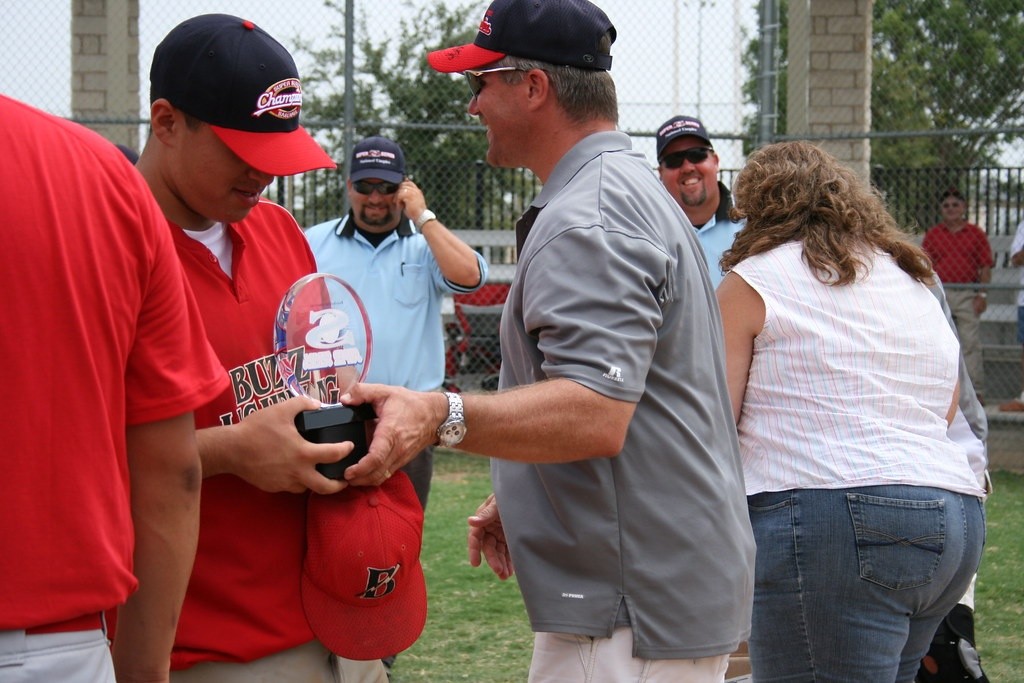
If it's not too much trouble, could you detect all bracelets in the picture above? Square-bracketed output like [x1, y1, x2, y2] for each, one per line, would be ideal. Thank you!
[978, 292, 988, 298]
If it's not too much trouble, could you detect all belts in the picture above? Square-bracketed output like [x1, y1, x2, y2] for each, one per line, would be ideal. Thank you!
[26, 610, 102, 634]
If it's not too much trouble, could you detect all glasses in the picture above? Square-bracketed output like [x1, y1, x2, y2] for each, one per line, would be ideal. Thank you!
[353, 179, 401, 195]
[942, 201, 963, 208]
[659, 146, 714, 169]
[462, 66, 553, 98]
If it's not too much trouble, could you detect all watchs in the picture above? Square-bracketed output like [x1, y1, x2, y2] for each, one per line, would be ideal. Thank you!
[434, 392, 466, 447]
[416, 211, 435, 233]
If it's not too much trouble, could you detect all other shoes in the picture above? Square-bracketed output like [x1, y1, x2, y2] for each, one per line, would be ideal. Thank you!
[1000, 398, 1024, 412]
[975, 394, 985, 406]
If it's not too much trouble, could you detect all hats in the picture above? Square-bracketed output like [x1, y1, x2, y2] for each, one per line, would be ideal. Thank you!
[939, 186, 967, 202]
[349, 136, 406, 187]
[151, 13, 338, 176]
[426, 0, 617, 73]
[299, 467, 426, 659]
[657, 115, 714, 160]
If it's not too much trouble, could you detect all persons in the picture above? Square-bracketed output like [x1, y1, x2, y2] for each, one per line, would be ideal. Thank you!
[0, 95, 229, 683]
[135, 14, 387, 683]
[1001, 220, 1024, 411]
[656, 115, 744, 290]
[338, 0, 754, 683]
[713, 140, 987, 682]
[304, 136, 489, 507]
[922, 188, 992, 404]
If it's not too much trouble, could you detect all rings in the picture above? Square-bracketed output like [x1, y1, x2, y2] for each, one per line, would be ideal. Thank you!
[405, 189, 407, 192]
[384, 471, 391, 478]
